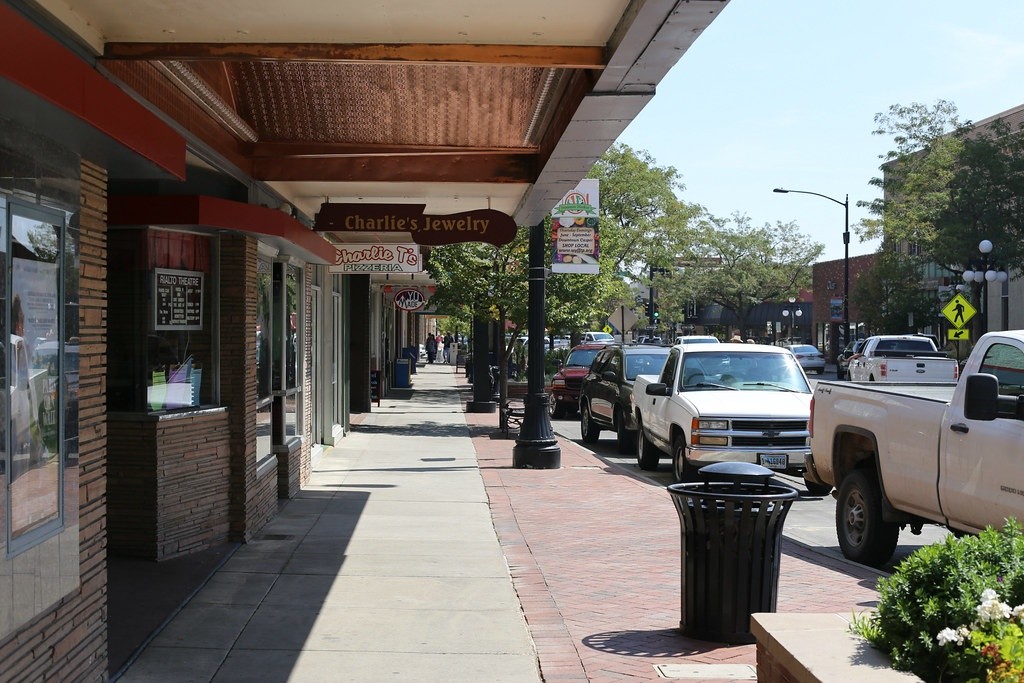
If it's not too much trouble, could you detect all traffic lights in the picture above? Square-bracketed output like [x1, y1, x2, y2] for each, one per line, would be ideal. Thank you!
[654, 311, 659, 317]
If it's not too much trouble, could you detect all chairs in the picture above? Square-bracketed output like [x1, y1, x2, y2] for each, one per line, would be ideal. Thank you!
[500, 389, 526, 438]
[456, 352, 469, 373]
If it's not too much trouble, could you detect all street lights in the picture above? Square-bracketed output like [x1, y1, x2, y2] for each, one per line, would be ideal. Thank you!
[773, 187, 851, 347]
[963, 240, 1009, 335]
[781, 297, 802, 345]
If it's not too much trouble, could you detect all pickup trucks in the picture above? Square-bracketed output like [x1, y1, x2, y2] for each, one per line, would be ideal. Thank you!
[807, 328, 1023, 571]
[629, 342, 834, 497]
[9, 337, 49, 464]
[846, 334, 959, 382]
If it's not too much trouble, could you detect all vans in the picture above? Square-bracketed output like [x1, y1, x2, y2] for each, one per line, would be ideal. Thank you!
[33, 345, 78, 417]
[787, 336, 802, 345]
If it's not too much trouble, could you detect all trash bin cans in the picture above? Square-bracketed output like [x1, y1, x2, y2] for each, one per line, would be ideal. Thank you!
[667, 462, 800, 644]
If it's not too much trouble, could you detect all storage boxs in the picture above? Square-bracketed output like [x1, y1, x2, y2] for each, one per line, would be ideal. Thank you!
[134, 368, 203, 412]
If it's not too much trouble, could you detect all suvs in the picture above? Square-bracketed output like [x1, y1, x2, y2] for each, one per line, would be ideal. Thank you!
[549, 341, 614, 419]
[578, 342, 676, 456]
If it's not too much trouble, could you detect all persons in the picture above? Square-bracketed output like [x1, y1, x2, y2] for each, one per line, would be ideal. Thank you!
[711, 329, 755, 363]
[10, 295, 55, 467]
[946, 340, 958, 358]
[426, 331, 453, 364]
[846, 353, 863, 363]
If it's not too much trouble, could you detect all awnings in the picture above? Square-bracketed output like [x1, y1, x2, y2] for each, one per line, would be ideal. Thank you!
[695, 299, 813, 326]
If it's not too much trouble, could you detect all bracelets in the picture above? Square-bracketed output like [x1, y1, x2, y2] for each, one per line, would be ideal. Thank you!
[849, 356, 853, 360]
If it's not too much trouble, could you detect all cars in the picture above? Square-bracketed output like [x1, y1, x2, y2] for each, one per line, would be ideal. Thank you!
[673, 335, 721, 344]
[635, 336, 662, 344]
[835, 341, 865, 379]
[457, 331, 616, 354]
[783, 344, 826, 374]
[769, 337, 786, 346]
